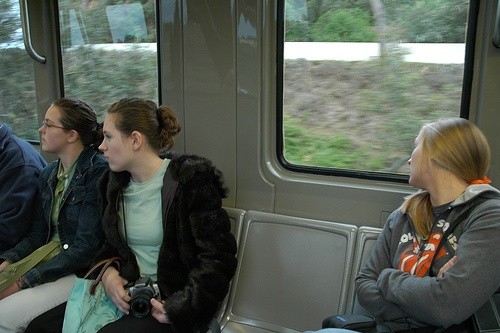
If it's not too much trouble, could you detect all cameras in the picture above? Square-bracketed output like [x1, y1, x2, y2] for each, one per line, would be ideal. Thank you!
[123, 277, 161, 318]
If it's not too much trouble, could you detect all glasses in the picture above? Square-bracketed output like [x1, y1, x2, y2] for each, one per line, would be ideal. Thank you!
[43, 120, 65, 129]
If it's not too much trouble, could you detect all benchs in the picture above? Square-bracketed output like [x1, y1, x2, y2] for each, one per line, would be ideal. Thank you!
[210, 207, 385, 333]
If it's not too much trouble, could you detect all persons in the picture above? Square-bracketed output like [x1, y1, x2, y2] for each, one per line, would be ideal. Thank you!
[0, 122, 47, 254]
[0, 99, 110, 333]
[25, 98, 237, 333]
[303, 116, 500, 333]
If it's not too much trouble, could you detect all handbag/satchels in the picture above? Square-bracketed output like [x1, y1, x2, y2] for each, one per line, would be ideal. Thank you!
[0, 241, 61, 292]
[62, 257, 124, 333]
[322, 313, 377, 333]
